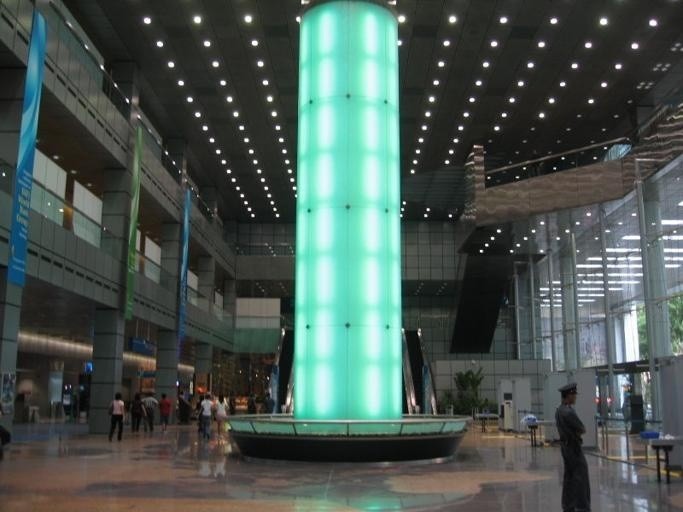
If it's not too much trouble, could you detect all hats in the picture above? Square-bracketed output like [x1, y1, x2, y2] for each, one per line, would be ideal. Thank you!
[556, 382, 580, 395]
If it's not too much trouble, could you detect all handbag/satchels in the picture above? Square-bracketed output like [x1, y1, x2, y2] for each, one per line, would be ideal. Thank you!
[107, 406, 114, 416]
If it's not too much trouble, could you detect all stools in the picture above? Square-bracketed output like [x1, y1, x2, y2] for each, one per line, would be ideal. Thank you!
[651, 440, 674, 484]
[528, 425, 538, 446]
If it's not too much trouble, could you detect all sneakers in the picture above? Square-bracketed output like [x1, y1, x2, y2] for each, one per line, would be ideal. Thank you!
[105, 429, 225, 444]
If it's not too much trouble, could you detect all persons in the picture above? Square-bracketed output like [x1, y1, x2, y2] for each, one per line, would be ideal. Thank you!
[175, 392, 236, 437]
[259, 393, 275, 412]
[156, 392, 171, 432]
[107, 391, 125, 442]
[246, 393, 257, 413]
[128, 392, 146, 435]
[141, 391, 158, 431]
[553, 382, 591, 511]
[0, 402, 10, 462]
